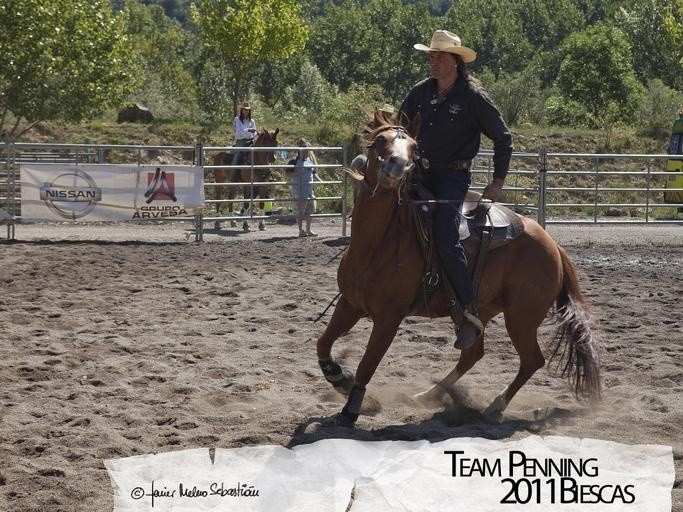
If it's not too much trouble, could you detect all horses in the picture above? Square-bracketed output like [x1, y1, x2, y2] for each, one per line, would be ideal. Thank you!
[316, 105, 603, 428]
[213, 127, 279, 232]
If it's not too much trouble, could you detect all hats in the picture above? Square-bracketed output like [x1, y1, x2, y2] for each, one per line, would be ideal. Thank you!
[239, 102, 251, 111]
[377, 104, 396, 115]
[412, 29, 476, 64]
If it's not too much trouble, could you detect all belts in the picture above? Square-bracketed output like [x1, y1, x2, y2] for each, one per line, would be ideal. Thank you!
[432, 157, 472, 170]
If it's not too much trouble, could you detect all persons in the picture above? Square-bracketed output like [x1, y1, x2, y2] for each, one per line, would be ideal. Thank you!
[383, 29, 511, 348]
[224, 101, 257, 188]
[286, 139, 322, 236]
[359, 103, 395, 137]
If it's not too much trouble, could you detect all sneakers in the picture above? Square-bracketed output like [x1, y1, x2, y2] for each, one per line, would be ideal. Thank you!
[452, 304, 483, 350]
